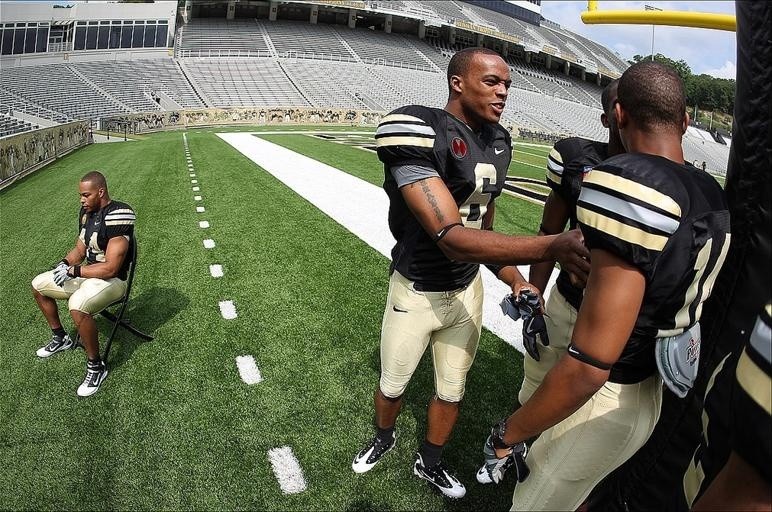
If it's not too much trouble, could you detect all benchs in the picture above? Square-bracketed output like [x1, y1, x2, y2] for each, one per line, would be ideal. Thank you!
[0, 0, 633, 144]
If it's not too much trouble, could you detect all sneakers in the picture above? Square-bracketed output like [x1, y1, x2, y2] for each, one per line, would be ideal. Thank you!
[352, 430, 396, 473]
[414, 452, 467, 499]
[477, 442, 529, 484]
[36, 335, 74, 358]
[77, 361, 108, 397]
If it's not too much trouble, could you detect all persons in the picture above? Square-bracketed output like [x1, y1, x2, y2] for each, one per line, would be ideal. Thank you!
[31, 172, 138, 400]
[350, 44, 590, 500]
[701, 161, 707, 171]
[692, 159, 699, 169]
[478, 61, 772, 512]
[150, 90, 155, 100]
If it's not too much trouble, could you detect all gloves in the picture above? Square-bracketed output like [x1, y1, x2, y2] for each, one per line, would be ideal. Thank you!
[498, 288, 550, 361]
[53, 262, 73, 288]
[484, 418, 528, 484]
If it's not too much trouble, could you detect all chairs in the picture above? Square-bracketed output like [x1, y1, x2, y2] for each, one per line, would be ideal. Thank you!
[70, 235, 158, 371]
[679, 125, 731, 176]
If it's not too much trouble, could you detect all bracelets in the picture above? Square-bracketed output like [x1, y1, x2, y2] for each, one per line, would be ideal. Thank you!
[59, 257, 71, 267]
[73, 265, 83, 278]
